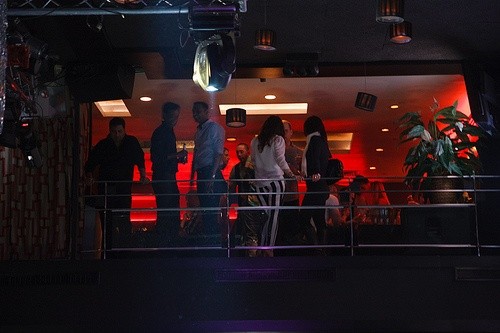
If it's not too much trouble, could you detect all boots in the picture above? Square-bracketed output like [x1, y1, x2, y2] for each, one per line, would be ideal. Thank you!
[306, 231, 319, 254]
[319, 229, 327, 252]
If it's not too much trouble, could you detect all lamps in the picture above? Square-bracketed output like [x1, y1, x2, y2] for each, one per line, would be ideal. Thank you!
[354, 49, 378, 112]
[226, 65, 246, 128]
[187, 0, 242, 91]
[389, 22, 412, 44]
[0, 31, 66, 151]
[253, 0, 277, 51]
[376, 0, 404, 26]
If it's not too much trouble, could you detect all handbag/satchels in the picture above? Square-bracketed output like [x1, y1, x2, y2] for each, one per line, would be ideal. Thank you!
[327, 159, 343, 184]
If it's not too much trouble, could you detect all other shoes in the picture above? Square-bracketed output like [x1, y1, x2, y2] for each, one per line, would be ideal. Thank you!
[256, 249, 274, 257]
[247, 239, 259, 257]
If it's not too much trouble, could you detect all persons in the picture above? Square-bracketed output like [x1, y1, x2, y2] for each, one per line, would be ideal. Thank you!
[324, 176, 418, 225]
[183, 101, 225, 239]
[218, 114, 332, 257]
[151, 103, 186, 236]
[82, 117, 145, 247]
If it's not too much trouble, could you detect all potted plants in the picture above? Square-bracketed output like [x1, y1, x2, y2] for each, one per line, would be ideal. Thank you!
[397, 97, 493, 204]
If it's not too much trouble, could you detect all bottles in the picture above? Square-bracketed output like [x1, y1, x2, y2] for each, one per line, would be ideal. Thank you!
[181, 143, 188, 163]
